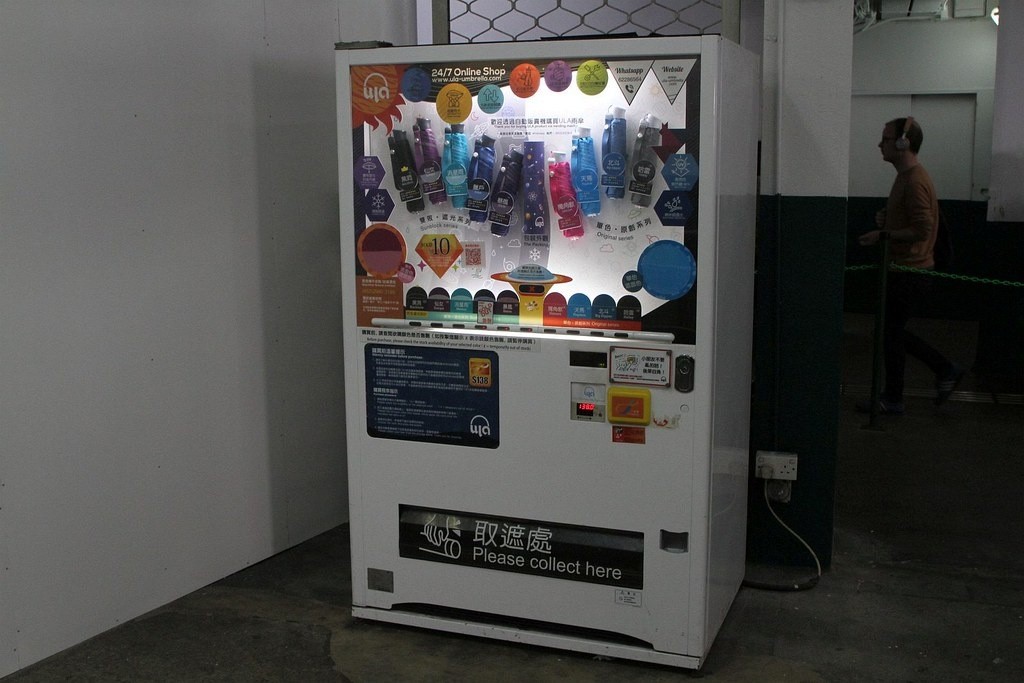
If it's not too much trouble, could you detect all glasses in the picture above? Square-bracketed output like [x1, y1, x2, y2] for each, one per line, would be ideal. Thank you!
[882, 135, 897, 143]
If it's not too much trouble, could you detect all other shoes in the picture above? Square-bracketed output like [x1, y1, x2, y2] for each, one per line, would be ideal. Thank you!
[869, 399, 905, 418]
[934, 367, 964, 402]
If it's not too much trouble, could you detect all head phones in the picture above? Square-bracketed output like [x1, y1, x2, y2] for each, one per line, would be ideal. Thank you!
[895, 116, 912, 151]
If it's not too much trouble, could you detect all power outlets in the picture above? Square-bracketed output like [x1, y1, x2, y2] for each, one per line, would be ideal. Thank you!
[755, 450, 797, 481]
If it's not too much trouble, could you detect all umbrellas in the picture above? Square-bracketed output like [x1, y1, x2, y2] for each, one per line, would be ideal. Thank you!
[388, 106, 663, 240]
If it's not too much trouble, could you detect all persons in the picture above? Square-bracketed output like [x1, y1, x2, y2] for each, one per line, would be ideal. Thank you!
[855, 116, 965, 415]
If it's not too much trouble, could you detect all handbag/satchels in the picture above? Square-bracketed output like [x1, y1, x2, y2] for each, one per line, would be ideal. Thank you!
[933, 208, 953, 273]
